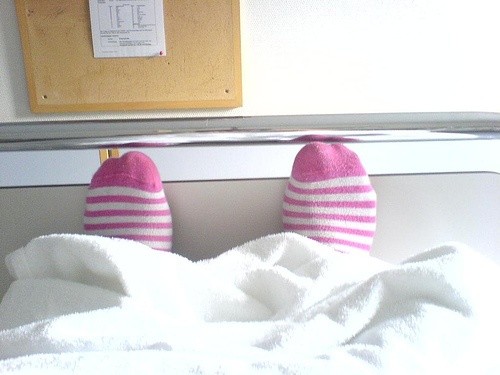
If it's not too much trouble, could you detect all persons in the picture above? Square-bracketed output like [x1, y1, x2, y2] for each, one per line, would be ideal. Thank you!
[79, 142, 380, 372]
